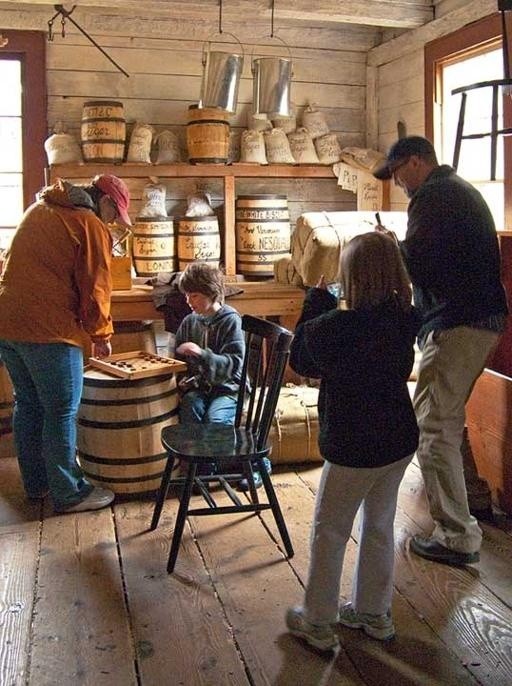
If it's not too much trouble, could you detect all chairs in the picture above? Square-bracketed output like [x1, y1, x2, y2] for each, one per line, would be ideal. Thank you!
[144, 313, 296, 579]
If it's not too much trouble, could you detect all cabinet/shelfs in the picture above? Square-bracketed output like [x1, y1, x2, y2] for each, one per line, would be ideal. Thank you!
[47, 162, 388, 326]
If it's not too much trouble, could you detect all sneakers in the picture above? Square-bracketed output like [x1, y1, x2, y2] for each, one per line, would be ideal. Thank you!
[285, 606, 338, 651]
[192, 462, 221, 494]
[237, 456, 273, 492]
[336, 601, 396, 641]
[22, 485, 117, 515]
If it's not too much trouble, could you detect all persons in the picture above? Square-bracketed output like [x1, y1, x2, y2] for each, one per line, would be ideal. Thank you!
[283, 231, 424, 653]
[168, 262, 276, 490]
[1, 173, 136, 515]
[372, 136, 509, 567]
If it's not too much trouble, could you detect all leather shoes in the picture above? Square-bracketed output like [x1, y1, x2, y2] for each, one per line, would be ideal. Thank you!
[408, 533, 479, 565]
[467, 506, 496, 524]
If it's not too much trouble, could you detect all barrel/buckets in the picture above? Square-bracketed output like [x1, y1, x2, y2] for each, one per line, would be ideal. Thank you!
[235, 194, 291, 276]
[187, 105, 229, 162]
[81, 101, 127, 162]
[79, 366, 182, 496]
[178, 215, 221, 272]
[199, 31, 245, 114]
[0, 360, 17, 433]
[133, 216, 177, 277]
[251, 35, 293, 120]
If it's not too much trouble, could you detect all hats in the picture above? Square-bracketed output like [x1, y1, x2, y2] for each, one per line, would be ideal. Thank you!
[371, 135, 434, 181]
[91, 171, 133, 228]
